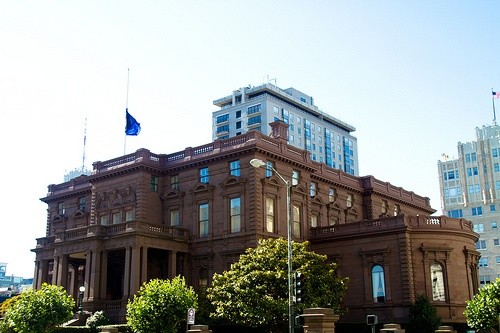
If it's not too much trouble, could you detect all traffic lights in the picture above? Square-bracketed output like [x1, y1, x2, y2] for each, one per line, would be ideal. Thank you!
[293, 273, 305, 303]
[367, 314, 376, 326]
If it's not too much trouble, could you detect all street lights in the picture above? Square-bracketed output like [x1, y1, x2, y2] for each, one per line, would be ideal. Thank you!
[250, 159, 295, 332]
[79, 286, 85, 312]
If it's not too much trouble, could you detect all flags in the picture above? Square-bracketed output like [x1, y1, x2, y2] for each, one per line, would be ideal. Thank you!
[492, 90, 500, 99]
[125, 108, 141, 136]
